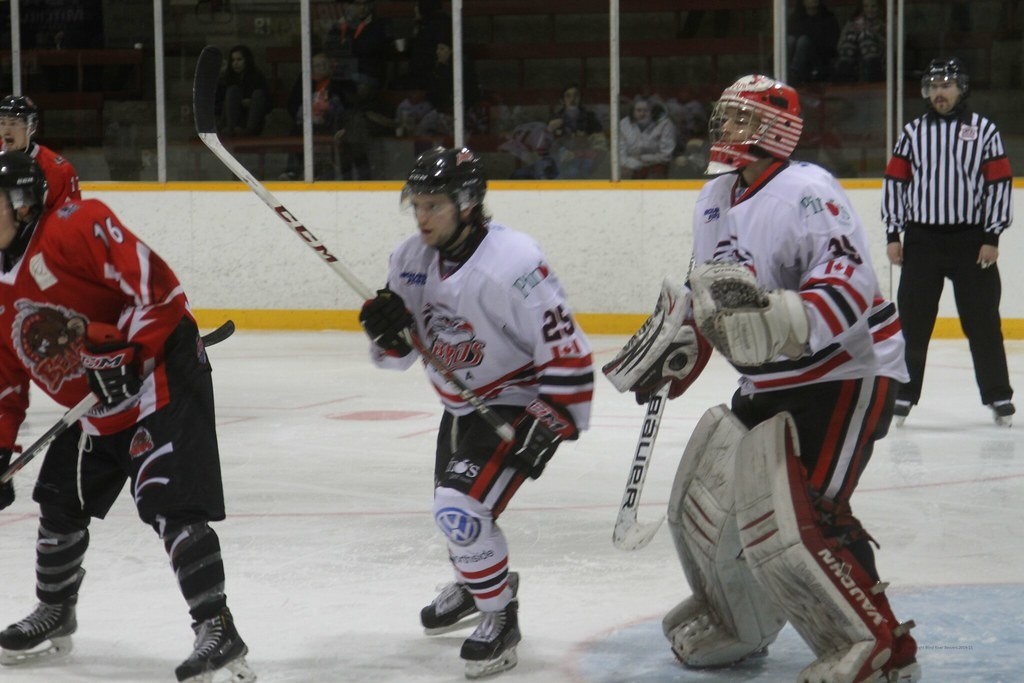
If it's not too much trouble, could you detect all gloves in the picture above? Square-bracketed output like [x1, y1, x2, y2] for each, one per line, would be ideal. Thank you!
[86, 356, 142, 409]
[692, 263, 809, 366]
[0, 447, 16, 510]
[358, 289, 414, 358]
[501, 395, 578, 479]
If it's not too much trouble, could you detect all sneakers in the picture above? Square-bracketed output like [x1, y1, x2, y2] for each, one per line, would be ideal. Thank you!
[459, 572, 522, 679]
[892, 399, 913, 426]
[417, 579, 482, 636]
[988, 400, 1016, 429]
[175, 607, 255, 682]
[0, 593, 79, 666]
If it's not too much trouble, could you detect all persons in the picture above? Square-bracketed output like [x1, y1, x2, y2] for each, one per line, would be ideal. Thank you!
[882, 57, 1016, 425]
[0, 95, 81, 511]
[0, 149, 255, 683]
[603, 78, 920, 683]
[216, 0, 886, 179]
[356, 143, 592, 680]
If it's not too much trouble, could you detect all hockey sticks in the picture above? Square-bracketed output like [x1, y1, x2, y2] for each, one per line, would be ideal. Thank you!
[0, 391, 99, 484]
[191, 42, 516, 440]
[612, 380, 670, 552]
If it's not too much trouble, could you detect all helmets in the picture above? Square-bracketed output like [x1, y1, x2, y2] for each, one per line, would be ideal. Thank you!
[0, 95, 38, 155]
[701, 74, 803, 175]
[920, 55, 970, 99]
[1, 148, 49, 272]
[406, 144, 487, 227]
